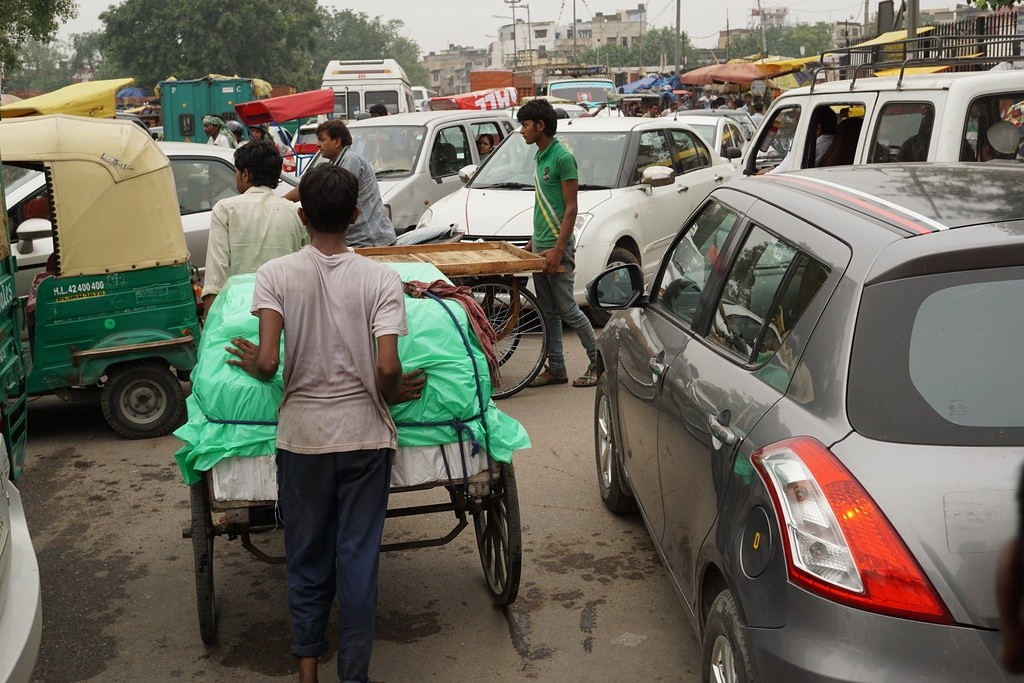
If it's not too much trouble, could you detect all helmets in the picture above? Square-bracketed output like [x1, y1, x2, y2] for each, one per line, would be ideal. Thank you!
[247, 123, 268, 135]
[226, 121, 244, 134]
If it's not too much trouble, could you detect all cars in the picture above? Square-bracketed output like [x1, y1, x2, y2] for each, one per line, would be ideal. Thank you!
[0, 72, 788, 682]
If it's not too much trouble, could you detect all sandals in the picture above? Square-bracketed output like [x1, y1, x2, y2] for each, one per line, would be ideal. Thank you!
[573, 366, 598, 386]
[525, 370, 568, 388]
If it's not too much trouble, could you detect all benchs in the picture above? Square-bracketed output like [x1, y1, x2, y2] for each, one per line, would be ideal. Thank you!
[26, 269, 59, 362]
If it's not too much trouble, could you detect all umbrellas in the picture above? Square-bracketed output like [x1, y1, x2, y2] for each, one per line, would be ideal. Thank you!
[681, 63, 765, 99]
[751, 54, 812, 88]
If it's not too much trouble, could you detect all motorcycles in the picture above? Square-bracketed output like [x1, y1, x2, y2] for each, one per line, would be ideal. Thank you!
[0, 115, 205, 433]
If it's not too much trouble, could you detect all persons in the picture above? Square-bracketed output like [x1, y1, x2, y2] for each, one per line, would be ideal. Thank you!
[514, 98, 600, 388]
[478, 134, 495, 164]
[755, 106, 839, 177]
[283, 120, 397, 249]
[199, 139, 310, 530]
[202, 111, 271, 151]
[978, 120, 1021, 163]
[354, 104, 411, 170]
[626, 88, 773, 126]
[223, 162, 431, 683]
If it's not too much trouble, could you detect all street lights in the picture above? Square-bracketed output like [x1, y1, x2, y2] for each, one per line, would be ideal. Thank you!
[491, 13, 528, 71]
[484, 33, 510, 69]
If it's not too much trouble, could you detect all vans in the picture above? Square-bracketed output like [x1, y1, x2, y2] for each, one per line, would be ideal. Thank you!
[317, 58, 417, 126]
[728, 33, 1024, 181]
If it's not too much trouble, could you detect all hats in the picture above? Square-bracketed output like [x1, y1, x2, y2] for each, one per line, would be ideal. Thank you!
[702, 84, 713, 92]
[987, 121, 1020, 154]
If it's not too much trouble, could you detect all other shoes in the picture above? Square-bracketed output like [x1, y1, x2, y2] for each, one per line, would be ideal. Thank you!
[216, 515, 226, 524]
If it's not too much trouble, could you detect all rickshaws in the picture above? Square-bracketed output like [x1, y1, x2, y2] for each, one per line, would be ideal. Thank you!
[180, 450, 524, 645]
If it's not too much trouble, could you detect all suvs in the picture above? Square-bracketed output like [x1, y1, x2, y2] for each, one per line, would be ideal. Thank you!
[584, 160, 1024, 683]
